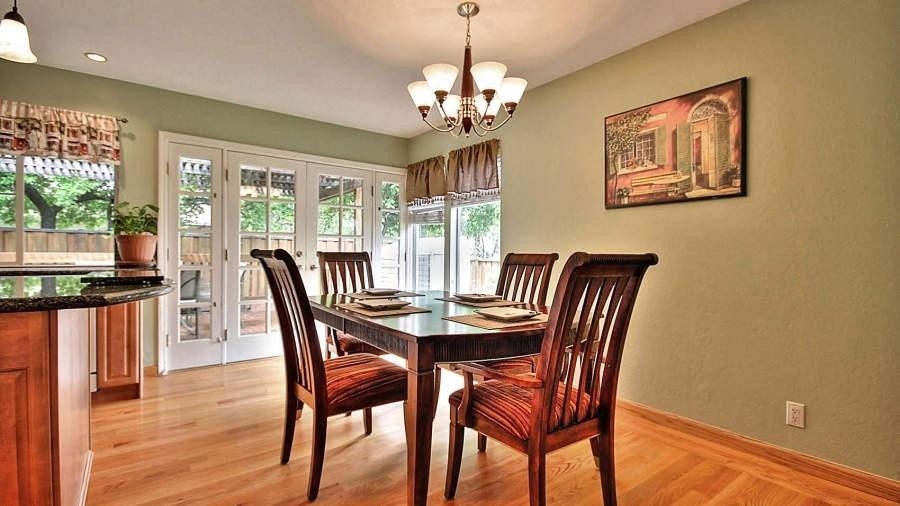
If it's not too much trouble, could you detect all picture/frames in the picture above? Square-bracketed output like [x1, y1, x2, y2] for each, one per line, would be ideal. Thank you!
[605, 76, 747, 209]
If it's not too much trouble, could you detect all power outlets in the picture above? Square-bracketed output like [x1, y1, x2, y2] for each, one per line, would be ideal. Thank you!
[786, 401, 805, 429]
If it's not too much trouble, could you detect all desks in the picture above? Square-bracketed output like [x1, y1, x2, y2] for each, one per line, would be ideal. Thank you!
[184, 253, 260, 329]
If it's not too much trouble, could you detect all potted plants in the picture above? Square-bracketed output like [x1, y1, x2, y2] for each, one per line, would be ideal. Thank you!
[108, 197, 159, 267]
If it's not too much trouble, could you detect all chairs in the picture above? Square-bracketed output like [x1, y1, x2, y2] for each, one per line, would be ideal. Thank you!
[444, 252, 659, 505]
[317, 252, 392, 416]
[433, 253, 559, 454]
[180, 269, 200, 336]
[251, 249, 408, 501]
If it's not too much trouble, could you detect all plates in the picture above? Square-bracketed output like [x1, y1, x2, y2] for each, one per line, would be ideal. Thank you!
[355, 298, 410, 310]
[361, 288, 402, 296]
[474, 306, 538, 322]
[453, 293, 503, 303]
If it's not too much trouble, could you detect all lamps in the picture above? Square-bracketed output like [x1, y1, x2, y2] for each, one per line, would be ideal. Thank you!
[408, 2, 528, 140]
[0, 1, 38, 66]
